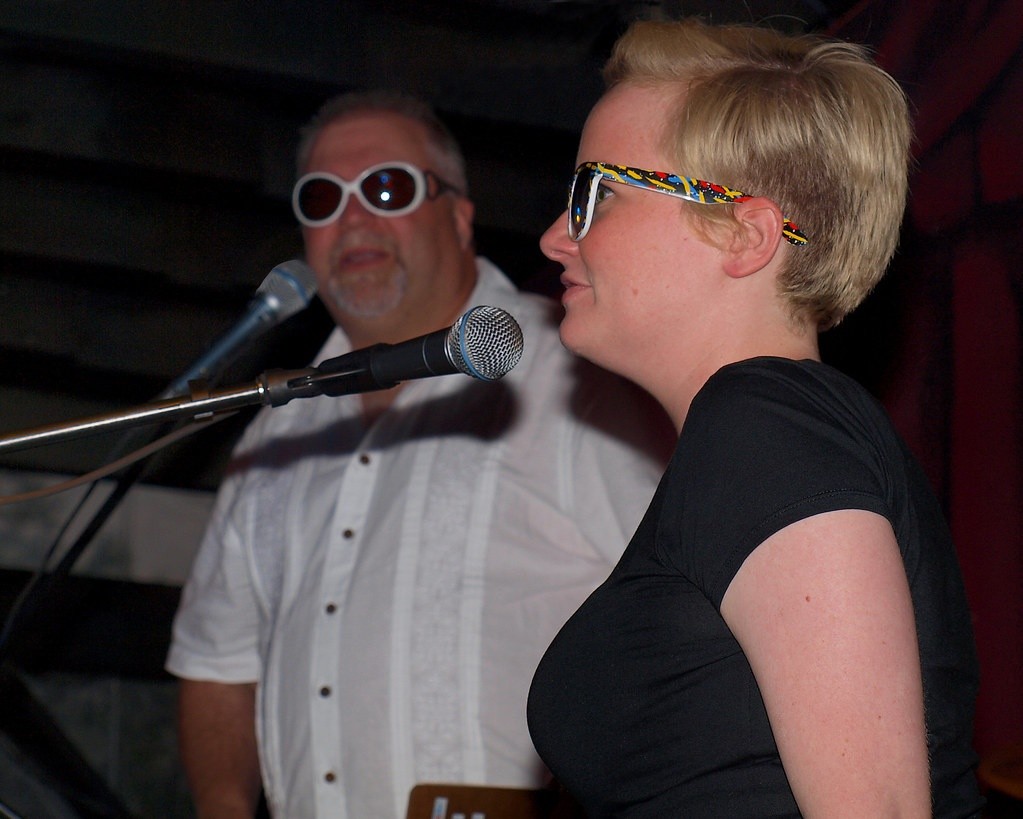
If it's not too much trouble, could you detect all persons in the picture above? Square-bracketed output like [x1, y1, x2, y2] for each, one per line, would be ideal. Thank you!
[525, 21, 981, 819]
[165, 90, 677, 819]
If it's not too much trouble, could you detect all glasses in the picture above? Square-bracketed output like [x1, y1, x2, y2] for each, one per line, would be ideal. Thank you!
[292, 161, 462, 228]
[567, 161, 808, 247]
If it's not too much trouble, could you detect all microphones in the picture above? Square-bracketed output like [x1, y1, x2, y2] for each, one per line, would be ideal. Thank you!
[128, 260, 313, 437]
[288, 305, 525, 399]
